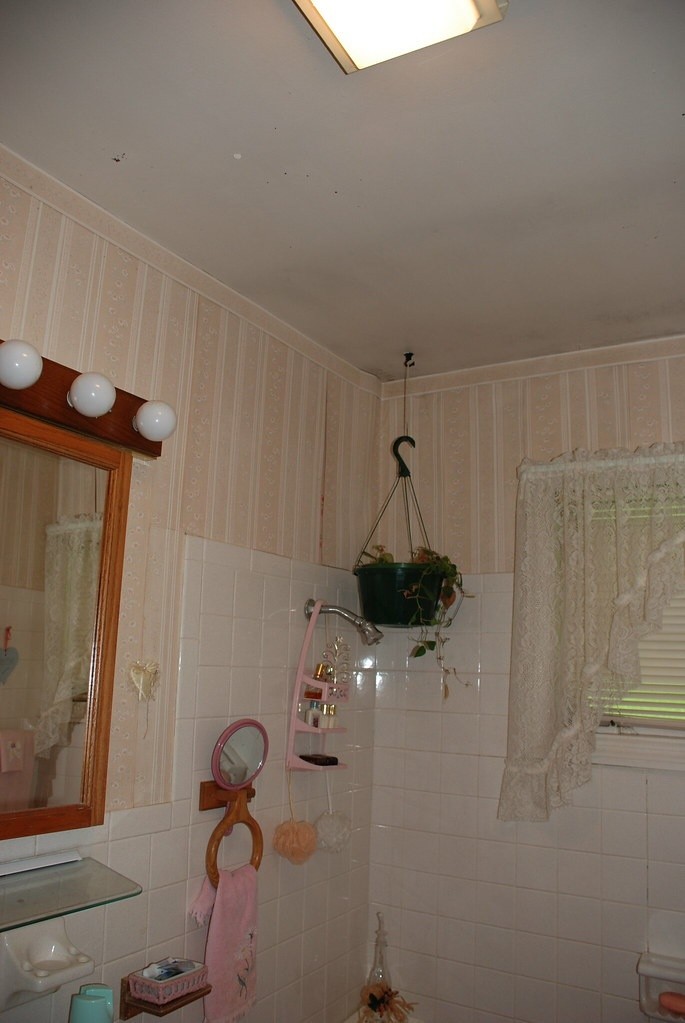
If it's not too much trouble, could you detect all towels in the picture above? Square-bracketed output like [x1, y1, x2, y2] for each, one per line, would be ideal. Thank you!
[185, 865, 260, 1022]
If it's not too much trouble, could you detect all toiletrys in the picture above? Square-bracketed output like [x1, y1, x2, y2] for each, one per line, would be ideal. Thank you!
[304, 661, 337, 730]
[142, 956, 178, 980]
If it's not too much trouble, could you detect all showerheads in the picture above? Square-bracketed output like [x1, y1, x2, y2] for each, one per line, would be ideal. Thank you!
[303, 597, 384, 646]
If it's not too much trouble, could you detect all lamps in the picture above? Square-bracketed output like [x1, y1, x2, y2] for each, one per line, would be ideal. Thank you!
[292, 1, 506, 76]
[132, 400, 178, 442]
[66, 371, 117, 417]
[0, 338, 44, 391]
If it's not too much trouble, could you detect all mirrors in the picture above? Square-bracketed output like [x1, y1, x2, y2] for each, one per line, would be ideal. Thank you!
[211, 719, 270, 837]
[1, 434, 134, 842]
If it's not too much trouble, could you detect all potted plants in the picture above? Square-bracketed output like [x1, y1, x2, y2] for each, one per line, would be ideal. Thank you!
[355, 546, 476, 701]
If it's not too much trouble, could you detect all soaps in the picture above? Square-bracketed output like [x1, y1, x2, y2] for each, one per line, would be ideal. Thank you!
[658, 990, 685, 1016]
